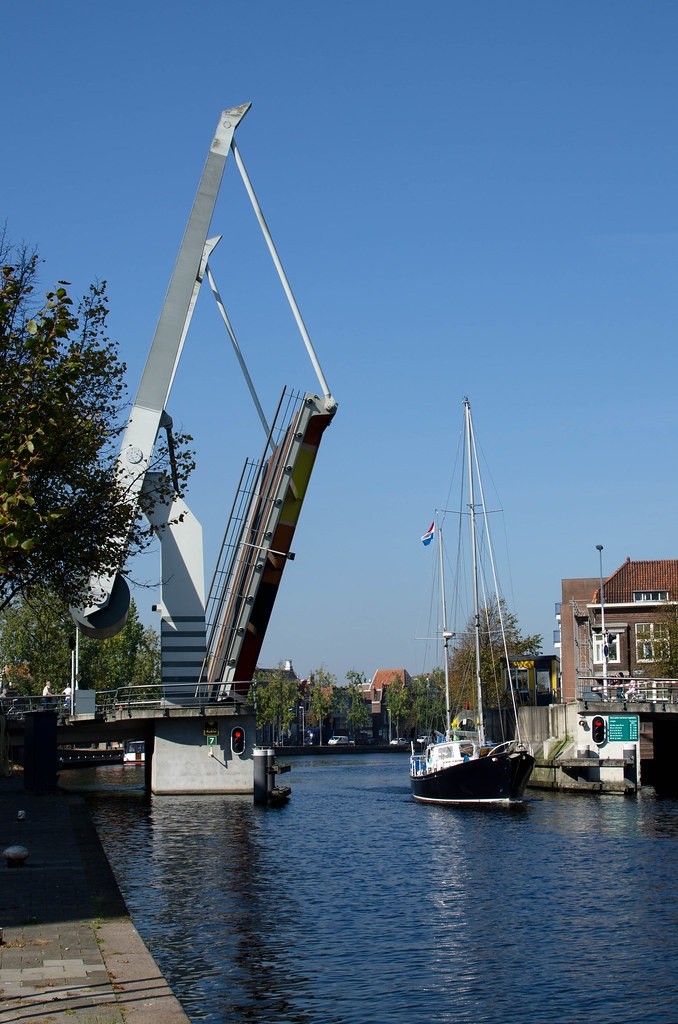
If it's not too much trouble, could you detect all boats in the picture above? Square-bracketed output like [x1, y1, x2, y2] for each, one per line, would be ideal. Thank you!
[123, 741, 145, 766]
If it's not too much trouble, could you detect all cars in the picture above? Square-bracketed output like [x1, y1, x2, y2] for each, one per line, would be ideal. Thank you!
[390, 737, 406, 747]
[275, 739, 291, 746]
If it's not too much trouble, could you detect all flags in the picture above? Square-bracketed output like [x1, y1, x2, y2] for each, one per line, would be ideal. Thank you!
[421, 521, 435, 546]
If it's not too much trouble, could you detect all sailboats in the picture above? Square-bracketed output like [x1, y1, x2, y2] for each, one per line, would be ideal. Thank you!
[408, 394, 537, 805]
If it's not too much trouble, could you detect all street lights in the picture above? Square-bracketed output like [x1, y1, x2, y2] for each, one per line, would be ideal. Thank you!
[300, 706, 304, 746]
[596, 545, 609, 703]
[387, 708, 391, 744]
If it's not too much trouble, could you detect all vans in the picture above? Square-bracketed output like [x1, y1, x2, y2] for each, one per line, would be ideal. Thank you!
[328, 736, 349, 746]
[417, 736, 432, 744]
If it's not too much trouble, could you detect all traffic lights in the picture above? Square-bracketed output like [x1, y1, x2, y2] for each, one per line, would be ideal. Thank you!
[592, 716, 606, 746]
[231, 726, 245, 755]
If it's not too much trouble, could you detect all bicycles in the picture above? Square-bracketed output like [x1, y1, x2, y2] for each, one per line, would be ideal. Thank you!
[5, 698, 23, 720]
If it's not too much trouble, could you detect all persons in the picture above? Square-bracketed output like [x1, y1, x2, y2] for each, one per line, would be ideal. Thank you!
[43, 682, 52, 709]
[0, 689, 7, 697]
[62, 683, 72, 708]
[614, 672, 624, 702]
[624, 676, 636, 702]
